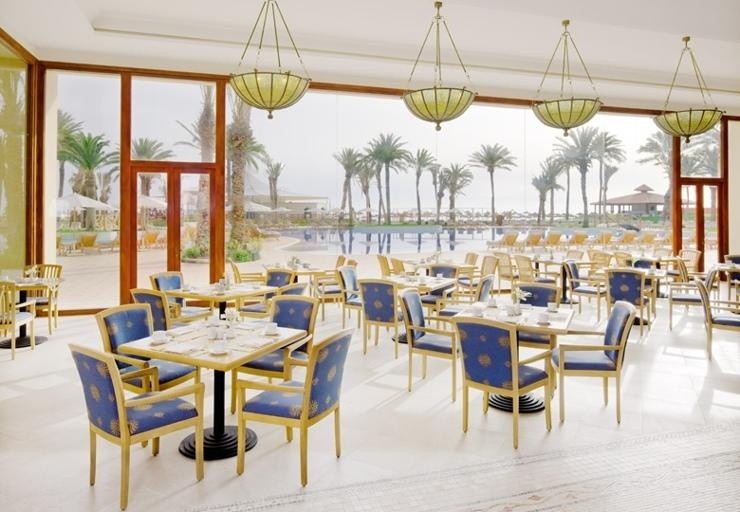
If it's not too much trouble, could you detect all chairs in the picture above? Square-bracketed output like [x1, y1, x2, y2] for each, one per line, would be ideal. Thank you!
[0, 263, 64, 360]
[359, 249, 739, 451]
[66, 250, 362, 512]
[486, 228, 676, 259]
[55, 230, 160, 253]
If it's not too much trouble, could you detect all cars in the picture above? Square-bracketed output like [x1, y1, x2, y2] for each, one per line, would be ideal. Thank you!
[151, 307, 279, 357]
[0, 276, 8, 281]
[472, 296, 561, 323]
[397, 270, 444, 283]
[647, 265, 655, 274]
[181, 278, 263, 293]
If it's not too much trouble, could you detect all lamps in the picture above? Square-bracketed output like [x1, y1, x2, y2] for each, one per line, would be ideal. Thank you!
[652, 34, 724, 145]
[0, 263, 64, 360]
[400, 1, 478, 133]
[55, 230, 160, 253]
[530, 18, 606, 136]
[228, 1, 313, 119]
[486, 228, 676, 259]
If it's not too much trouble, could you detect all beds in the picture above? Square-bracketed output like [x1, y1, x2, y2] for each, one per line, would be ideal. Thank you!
[56, 189, 294, 230]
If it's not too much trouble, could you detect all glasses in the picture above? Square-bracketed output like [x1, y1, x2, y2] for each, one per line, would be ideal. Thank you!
[400, 1, 478, 133]
[530, 18, 606, 136]
[652, 34, 724, 145]
[228, 1, 313, 119]
